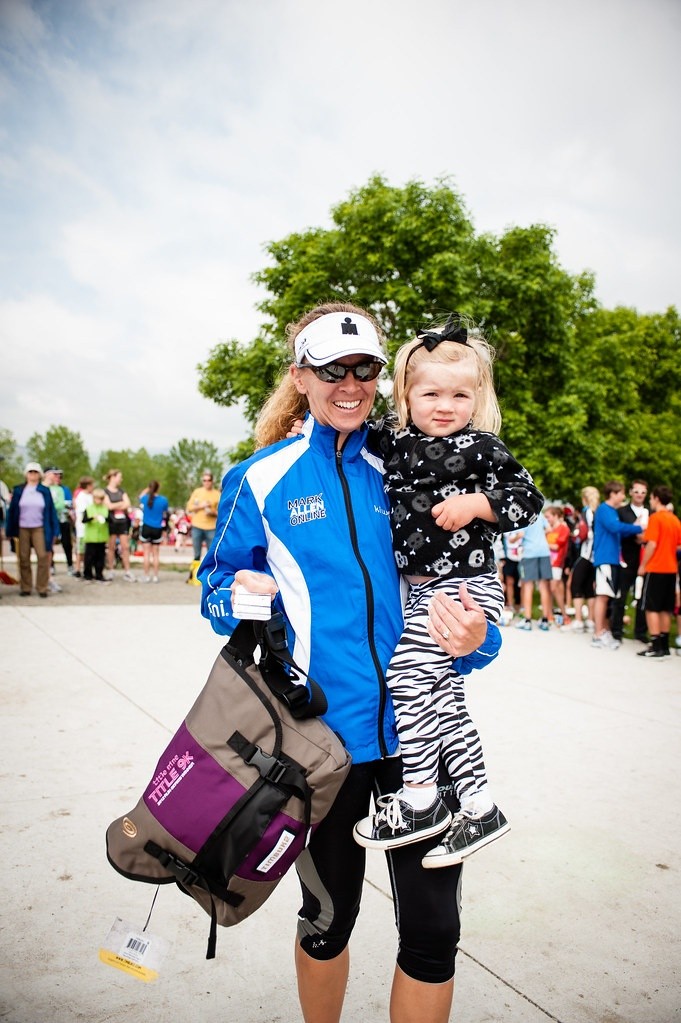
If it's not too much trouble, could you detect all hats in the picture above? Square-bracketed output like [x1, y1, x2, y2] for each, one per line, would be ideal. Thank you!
[44, 465, 62, 473]
[26, 462, 43, 475]
[294, 312, 388, 367]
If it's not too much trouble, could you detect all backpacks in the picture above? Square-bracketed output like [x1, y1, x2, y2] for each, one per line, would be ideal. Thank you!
[186, 561, 202, 586]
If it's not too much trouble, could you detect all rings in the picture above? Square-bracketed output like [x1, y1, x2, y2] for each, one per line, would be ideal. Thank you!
[442, 630, 450, 640]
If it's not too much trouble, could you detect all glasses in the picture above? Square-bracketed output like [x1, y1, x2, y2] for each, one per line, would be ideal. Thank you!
[299, 361, 385, 383]
[633, 489, 647, 493]
[203, 479, 211, 482]
[28, 470, 38, 473]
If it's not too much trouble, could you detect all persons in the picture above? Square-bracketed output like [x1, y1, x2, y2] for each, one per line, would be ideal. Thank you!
[0, 463, 221, 598]
[494, 479, 681, 662]
[286, 319, 545, 868]
[197, 301, 501, 1023]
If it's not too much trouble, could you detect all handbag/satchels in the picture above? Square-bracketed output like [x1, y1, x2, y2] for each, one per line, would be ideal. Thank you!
[106, 603, 352, 928]
[111, 510, 126, 524]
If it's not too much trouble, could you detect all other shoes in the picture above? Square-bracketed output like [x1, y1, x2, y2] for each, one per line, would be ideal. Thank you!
[505, 606, 573, 615]
[49, 582, 61, 593]
[638, 637, 649, 643]
[613, 635, 621, 641]
[145, 576, 158, 583]
[636, 650, 672, 661]
[103, 572, 114, 580]
[81, 577, 110, 585]
[563, 617, 572, 625]
[18, 591, 48, 598]
[51, 568, 56, 575]
[124, 574, 138, 582]
[76, 570, 81, 578]
[67, 566, 75, 576]
[676, 638, 681, 647]
[562, 620, 594, 633]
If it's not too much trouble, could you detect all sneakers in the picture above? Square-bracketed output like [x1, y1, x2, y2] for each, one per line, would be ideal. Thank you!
[353, 797, 453, 851]
[516, 619, 548, 630]
[422, 802, 511, 869]
[592, 633, 620, 650]
